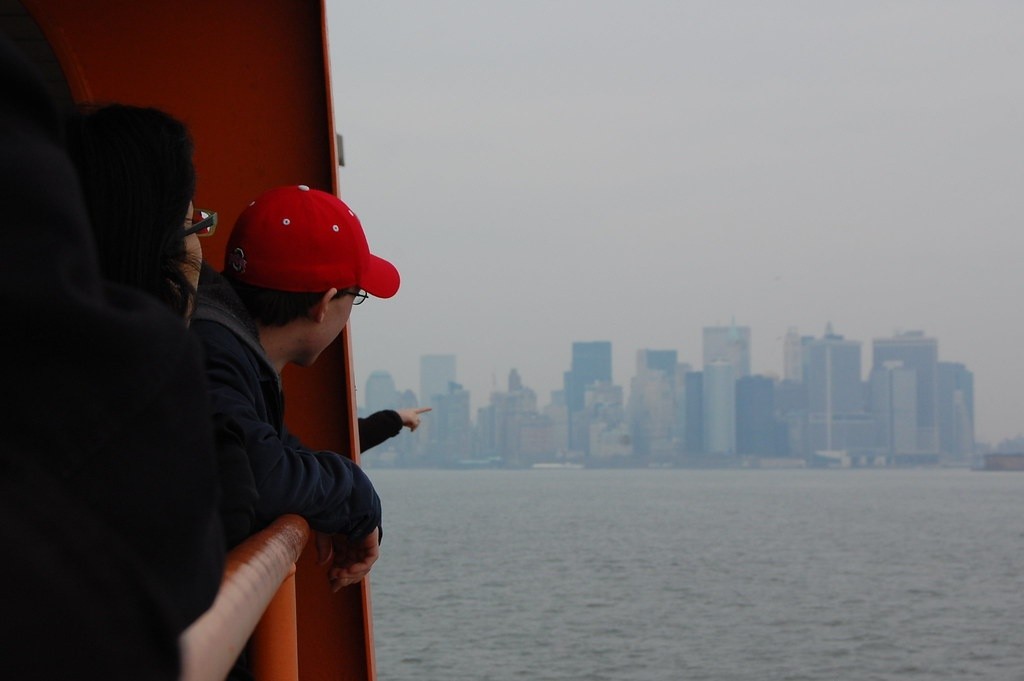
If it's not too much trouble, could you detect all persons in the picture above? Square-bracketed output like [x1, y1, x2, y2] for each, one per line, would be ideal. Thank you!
[0, 36, 433, 680]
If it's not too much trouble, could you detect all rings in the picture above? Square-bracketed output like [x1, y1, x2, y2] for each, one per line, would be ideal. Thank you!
[417, 418, 421, 421]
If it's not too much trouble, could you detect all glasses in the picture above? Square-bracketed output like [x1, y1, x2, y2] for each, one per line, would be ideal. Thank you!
[314, 288, 369, 305]
[182, 209, 217, 239]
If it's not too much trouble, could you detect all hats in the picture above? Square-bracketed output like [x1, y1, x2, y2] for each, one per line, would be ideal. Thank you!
[223, 185, 401, 298]
